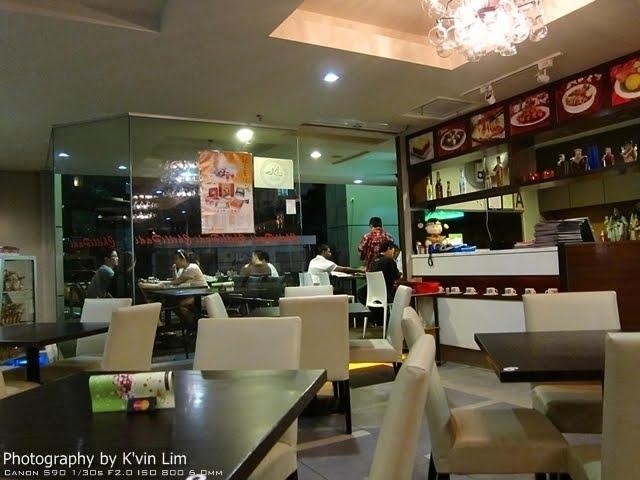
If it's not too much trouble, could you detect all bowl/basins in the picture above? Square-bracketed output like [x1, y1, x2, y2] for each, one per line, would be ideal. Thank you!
[562, 83, 596, 114]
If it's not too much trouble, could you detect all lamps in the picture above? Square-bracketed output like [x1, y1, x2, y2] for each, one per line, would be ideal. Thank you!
[459, 52, 561, 104]
[418, 0, 548, 63]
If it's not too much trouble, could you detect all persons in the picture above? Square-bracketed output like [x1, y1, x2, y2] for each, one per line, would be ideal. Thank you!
[239, 252, 280, 278]
[425, 218, 446, 246]
[569, 148, 591, 178]
[601, 147, 616, 168]
[358, 241, 407, 325]
[91, 247, 120, 297]
[372, 240, 402, 296]
[163, 246, 210, 335]
[240, 249, 271, 279]
[109, 249, 166, 330]
[308, 244, 365, 286]
[557, 154, 569, 178]
[356, 216, 395, 273]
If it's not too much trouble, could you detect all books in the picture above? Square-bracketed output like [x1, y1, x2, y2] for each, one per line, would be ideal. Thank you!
[533, 216, 596, 248]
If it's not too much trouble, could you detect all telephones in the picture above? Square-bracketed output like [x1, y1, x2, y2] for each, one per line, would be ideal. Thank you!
[429, 242, 443, 253]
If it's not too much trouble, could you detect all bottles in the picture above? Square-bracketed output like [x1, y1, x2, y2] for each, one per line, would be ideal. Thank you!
[556, 140, 638, 178]
[426, 169, 466, 201]
[482, 155, 492, 189]
[495, 156, 503, 187]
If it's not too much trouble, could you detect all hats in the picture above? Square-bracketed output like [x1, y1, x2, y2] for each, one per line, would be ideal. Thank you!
[369, 217, 383, 226]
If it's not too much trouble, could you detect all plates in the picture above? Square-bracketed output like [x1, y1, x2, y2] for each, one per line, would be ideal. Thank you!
[440, 128, 467, 151]
[614, 78, 640, 99]
[511, 106, 550, 126]
[452, 292, 517, 297]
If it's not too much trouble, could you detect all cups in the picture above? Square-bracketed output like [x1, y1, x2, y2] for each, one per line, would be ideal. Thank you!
[439, 286, 559, 294]
